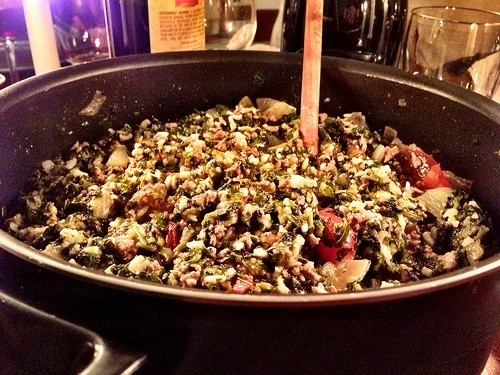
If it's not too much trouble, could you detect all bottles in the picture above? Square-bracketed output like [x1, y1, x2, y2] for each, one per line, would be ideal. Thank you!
[279, 0, 408, 68]
[46, 0, 149, 72]
[107, 1, 207, 59]
[1, 0, 37, 89]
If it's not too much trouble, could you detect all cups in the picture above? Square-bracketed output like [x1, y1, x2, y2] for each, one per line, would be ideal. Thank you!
[393, 4, 500, 105]
[203, 0, 259, 50]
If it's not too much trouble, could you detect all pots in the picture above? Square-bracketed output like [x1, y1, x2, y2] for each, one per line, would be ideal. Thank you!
[0, 48, 500, 375]
